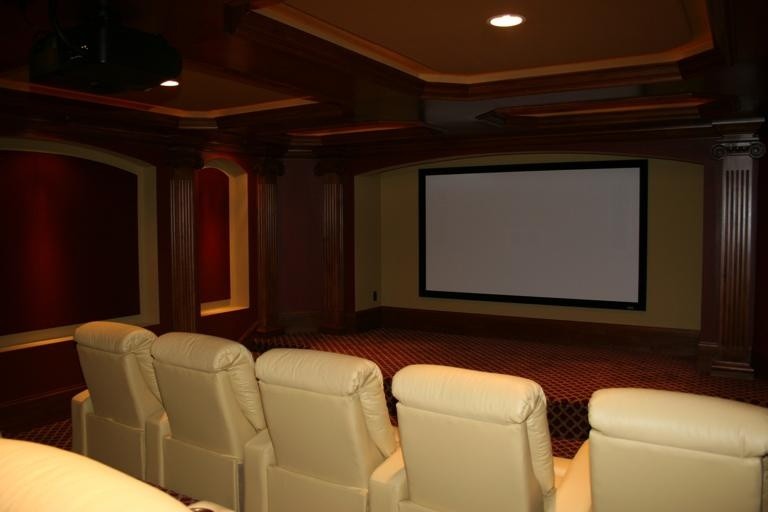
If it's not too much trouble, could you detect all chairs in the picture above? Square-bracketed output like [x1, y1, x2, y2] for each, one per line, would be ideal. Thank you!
[70, 320, 160, 487]
[549, 384, 767, 512]
[367, 359, 573, 512]
[149, 336, 268, 510]
[236, 343, 408, 512]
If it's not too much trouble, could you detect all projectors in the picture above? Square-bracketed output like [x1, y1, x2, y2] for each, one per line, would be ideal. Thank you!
[28, 22, 183, 95]
